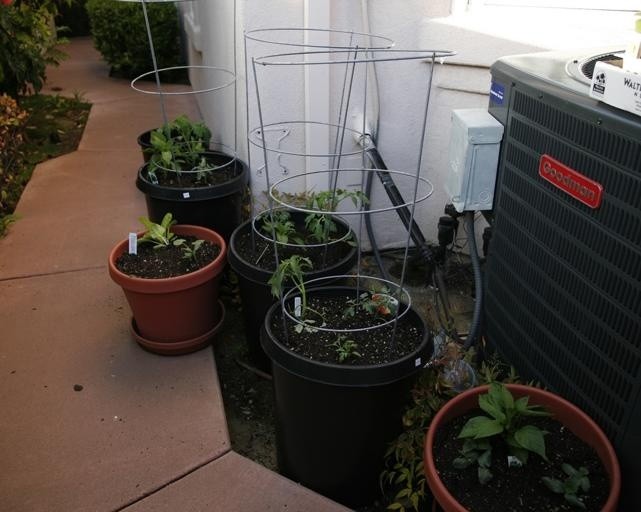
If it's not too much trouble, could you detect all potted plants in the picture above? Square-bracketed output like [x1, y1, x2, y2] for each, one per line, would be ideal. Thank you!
[259, 287, 434, 508]
[136, 115, 249, 241]
[229, 207, 362, 330]
[107, 213, 228, 356]
[423, 377, 620, 508]
[135, 113, 212, 163]
